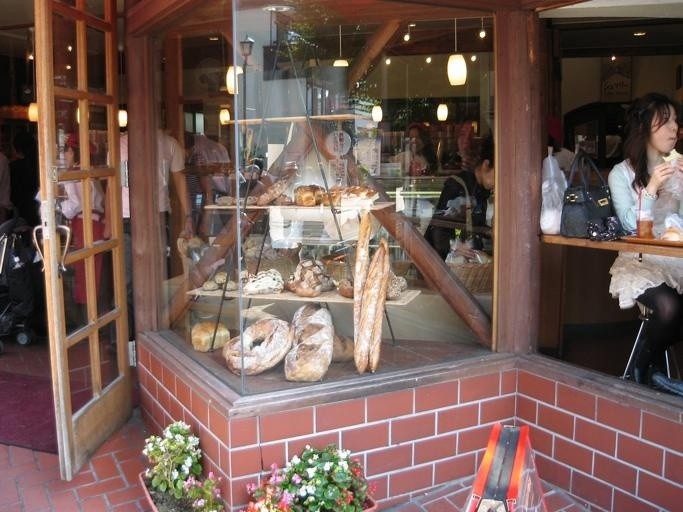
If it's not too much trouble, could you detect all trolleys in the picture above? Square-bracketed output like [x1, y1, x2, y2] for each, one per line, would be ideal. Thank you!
[0, 216, 37, 353]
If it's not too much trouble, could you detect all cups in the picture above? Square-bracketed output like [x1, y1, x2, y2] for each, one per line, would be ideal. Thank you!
[635, 208, 654, 239]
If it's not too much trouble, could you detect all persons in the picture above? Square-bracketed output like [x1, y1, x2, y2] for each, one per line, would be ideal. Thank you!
[411, 134, 495, 287]
[604, 90, 683, 393]
[445, 117, 492, 172]
[391, 119, 438, 176]
[1, 103, 232, 353]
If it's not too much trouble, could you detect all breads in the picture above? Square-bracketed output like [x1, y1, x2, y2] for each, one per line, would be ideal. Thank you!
[660, 227, 683, 242]
[191, 178, 408, 382]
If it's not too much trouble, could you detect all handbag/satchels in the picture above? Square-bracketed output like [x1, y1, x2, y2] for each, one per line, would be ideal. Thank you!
[540, 151, 622, 241]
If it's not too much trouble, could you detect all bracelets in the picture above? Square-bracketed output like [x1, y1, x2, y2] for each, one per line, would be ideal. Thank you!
[641, 188, 659, 200]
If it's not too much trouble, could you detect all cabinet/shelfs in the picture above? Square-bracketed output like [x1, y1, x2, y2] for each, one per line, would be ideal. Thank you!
[187, 18, 420, 353]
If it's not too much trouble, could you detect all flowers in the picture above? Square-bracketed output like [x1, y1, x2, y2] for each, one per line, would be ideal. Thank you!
[239, 444, 377, 512]
[141, 418, 223, 512]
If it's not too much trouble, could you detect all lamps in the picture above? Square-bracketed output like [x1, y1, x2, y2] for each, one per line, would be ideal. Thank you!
[225, 65, 243, 95]
[219, 109, 230, 125]
[372, 106, 383, 121]
[28, 28, 38, 121]
[479, 18, 486, 38]
[436, 97, 449, 121]
[333, 25, 348, 66]
[446, 19, 467, 86]
[77, 107, 89, 123]
[118, 41, 128, 126]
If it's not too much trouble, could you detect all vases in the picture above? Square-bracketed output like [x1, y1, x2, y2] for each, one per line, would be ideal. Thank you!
[363, 491, 377, 512]
[138, 470, 226, 512]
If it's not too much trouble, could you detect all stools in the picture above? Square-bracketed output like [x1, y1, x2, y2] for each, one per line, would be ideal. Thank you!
[619, 299, 680, 382]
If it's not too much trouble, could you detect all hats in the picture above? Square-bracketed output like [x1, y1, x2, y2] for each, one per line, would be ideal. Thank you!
[66, 134, 96, 155]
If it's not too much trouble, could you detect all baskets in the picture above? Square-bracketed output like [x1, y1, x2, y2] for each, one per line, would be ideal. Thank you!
[447, 263, 493, 295]
